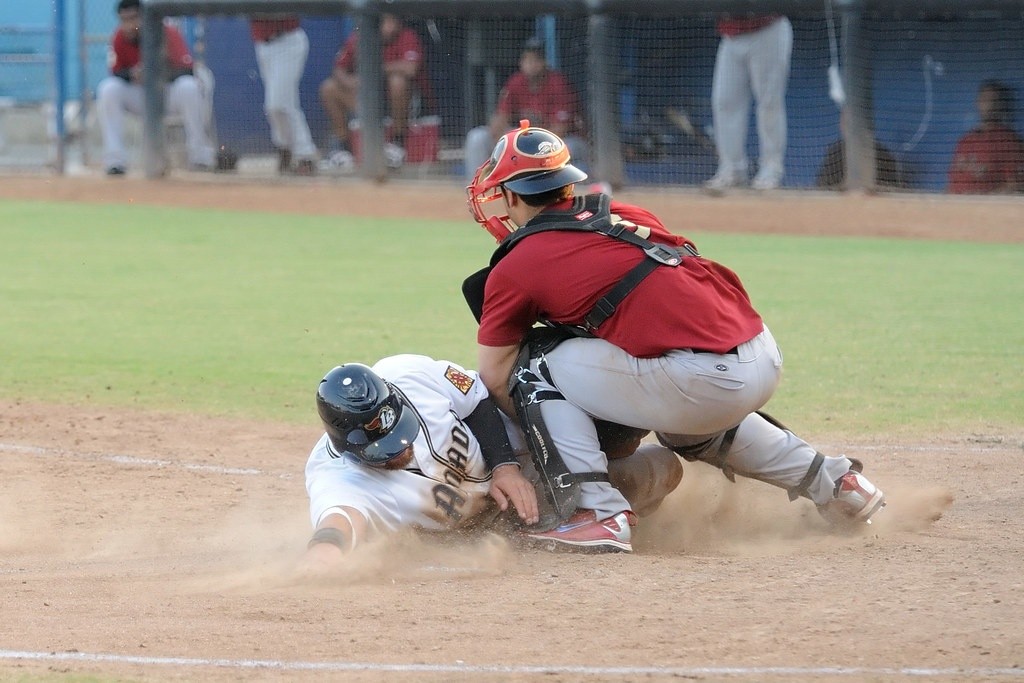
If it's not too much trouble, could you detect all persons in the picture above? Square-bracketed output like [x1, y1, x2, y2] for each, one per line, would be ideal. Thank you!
[465, 39, 588, 184]
[248, 1, 322, 176]
[819, 112, 897, 187]
[296, 353, 684, 584]
[946, 82, 1024, 195]
[465, 119, 886, 554]
[701, 0, 794, 195]
[95, 0, 220, 175]
[319, 1, 424, 168]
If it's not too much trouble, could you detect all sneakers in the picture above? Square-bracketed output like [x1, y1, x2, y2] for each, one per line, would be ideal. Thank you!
[518, 508, 638, 553]
[817, 469, 887, 528]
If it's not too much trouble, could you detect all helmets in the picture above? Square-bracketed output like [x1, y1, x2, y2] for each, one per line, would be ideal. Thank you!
[465, 119, 589, 243]
[316, 363, 420, 463]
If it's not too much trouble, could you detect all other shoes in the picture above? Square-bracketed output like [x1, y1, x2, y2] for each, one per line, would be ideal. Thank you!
[278, 151, 313, 176]
[751, 177, 784, 187]
